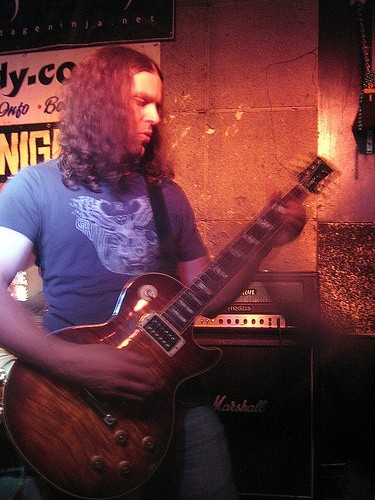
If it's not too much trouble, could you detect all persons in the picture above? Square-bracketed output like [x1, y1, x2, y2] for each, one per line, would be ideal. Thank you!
[0, 45, 312, 500]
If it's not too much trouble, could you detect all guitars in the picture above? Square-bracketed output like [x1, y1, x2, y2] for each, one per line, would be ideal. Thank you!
[3, 151, 339, 500]
